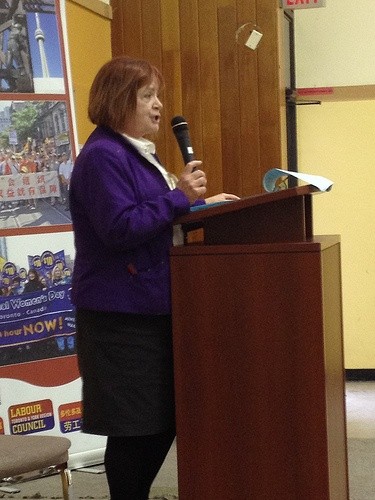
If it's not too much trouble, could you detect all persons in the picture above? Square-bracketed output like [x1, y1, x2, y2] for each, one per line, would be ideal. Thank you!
[67, 56, 244, 500]
[0, 139, 82, 367]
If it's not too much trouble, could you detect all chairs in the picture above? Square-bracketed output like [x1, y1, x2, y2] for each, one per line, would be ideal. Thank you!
[0, 430, 74, 500]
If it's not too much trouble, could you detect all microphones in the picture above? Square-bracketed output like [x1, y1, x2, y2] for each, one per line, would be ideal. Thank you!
[170, 115, 197, 172]
